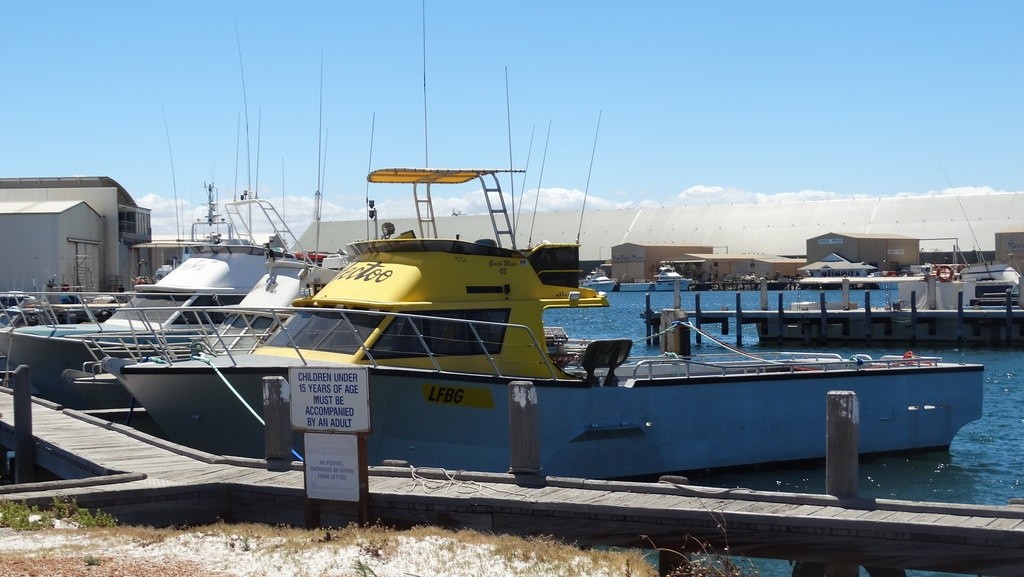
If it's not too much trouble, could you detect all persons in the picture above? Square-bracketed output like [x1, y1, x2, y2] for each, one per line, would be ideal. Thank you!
[58, 284, 80, 304]
[118, 285, 125, 303]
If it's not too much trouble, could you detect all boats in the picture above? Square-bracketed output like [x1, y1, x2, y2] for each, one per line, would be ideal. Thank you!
[757, 263, 1024, 341]
[583, 264, 693, 292]
[3, 3, 398, 404]
[82, 1, 984, 480]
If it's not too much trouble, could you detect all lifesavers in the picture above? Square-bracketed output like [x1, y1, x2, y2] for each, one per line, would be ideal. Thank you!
[936, 266, 955, 282]
